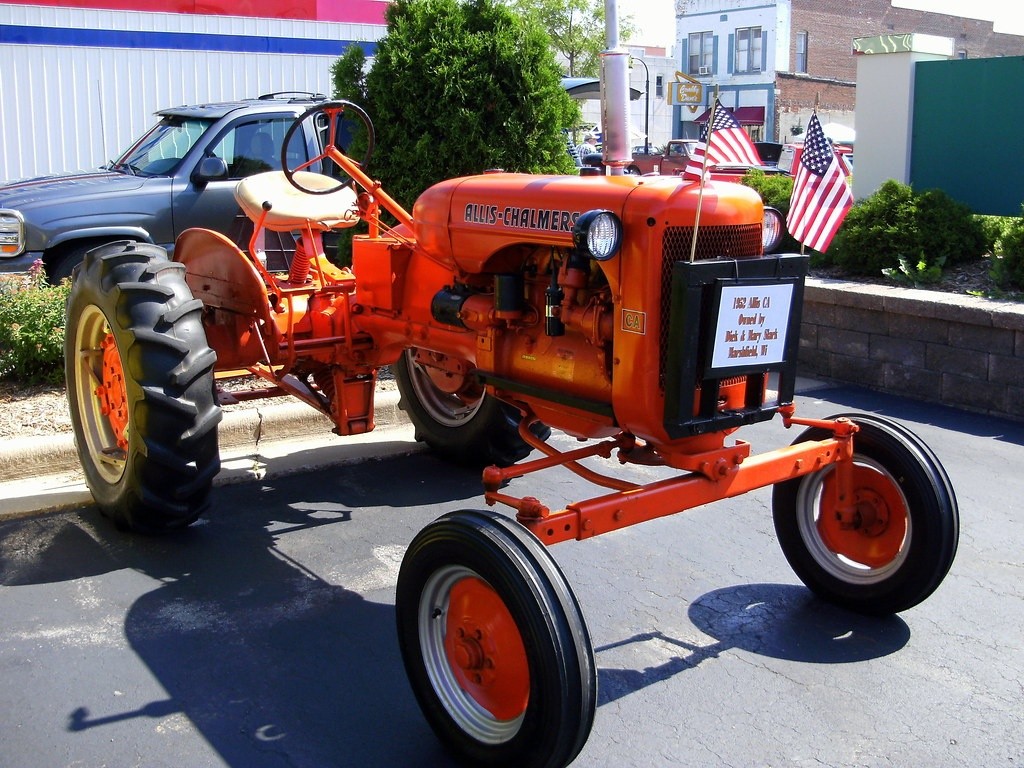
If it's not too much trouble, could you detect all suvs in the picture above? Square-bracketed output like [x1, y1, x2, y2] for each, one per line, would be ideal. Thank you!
[0, 89, 357, 289]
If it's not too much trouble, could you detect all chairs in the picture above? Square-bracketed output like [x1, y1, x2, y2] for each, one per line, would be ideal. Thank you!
[234, 132, 276, 165]
[233, 169, 361, 234]
[283, 133, 306, 171]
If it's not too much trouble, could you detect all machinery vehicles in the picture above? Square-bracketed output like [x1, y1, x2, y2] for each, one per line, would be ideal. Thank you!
[57, 100, 964, 768]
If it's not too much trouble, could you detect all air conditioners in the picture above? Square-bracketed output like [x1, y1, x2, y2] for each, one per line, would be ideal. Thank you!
[699, 66, 709, 75]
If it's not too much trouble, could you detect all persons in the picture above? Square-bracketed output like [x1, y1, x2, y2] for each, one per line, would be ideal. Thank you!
[575, 133, 600, 167]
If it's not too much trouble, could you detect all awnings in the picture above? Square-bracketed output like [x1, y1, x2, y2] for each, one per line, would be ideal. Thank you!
[694, 106, 764, 126]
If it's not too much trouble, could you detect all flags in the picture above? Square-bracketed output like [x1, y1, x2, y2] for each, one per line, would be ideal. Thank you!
[681, 98, 765, 182]
[786, 110, 854, 254]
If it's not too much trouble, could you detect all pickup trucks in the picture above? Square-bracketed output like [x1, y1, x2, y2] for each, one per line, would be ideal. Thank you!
[703, 139, 853, 214]
[627, 138, 698, 177]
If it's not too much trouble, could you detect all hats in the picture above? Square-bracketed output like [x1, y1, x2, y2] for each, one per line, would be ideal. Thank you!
[584, 132, 600, 139]
